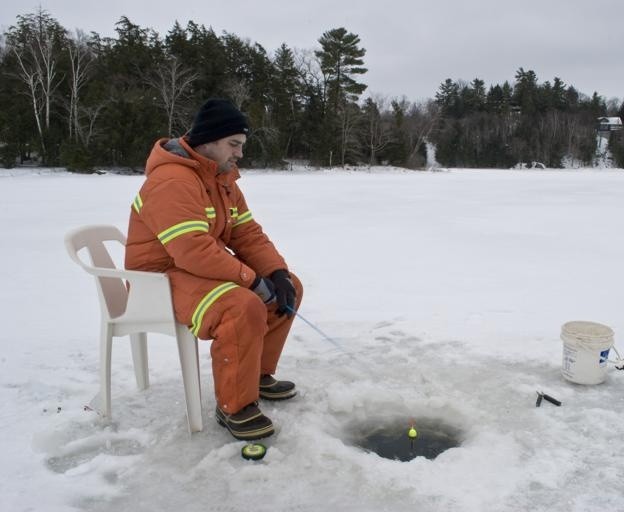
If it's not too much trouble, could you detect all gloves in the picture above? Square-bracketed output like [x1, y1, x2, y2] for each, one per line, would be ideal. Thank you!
[248, 269, 298, 321]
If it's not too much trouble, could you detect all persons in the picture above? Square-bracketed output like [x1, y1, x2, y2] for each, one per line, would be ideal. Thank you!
[123, 98, 305, 443]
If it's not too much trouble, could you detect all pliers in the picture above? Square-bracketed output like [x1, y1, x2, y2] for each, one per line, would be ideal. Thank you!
[535, 391, 562, 407]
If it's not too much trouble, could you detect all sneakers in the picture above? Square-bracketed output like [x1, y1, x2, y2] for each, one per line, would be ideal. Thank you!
[259, 373, 297, 403]
[215, 399, 275, 442]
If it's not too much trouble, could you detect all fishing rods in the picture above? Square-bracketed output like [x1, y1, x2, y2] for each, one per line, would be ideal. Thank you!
[281, 302, 419, 438]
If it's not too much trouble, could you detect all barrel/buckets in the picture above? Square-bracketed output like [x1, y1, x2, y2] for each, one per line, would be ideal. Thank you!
[560, 321, 614, 385]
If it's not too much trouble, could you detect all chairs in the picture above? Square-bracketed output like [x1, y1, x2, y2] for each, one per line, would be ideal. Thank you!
[64, 223, 201, 431]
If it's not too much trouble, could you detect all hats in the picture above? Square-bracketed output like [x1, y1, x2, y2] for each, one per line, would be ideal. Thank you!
[187, 98, 250, 149]
